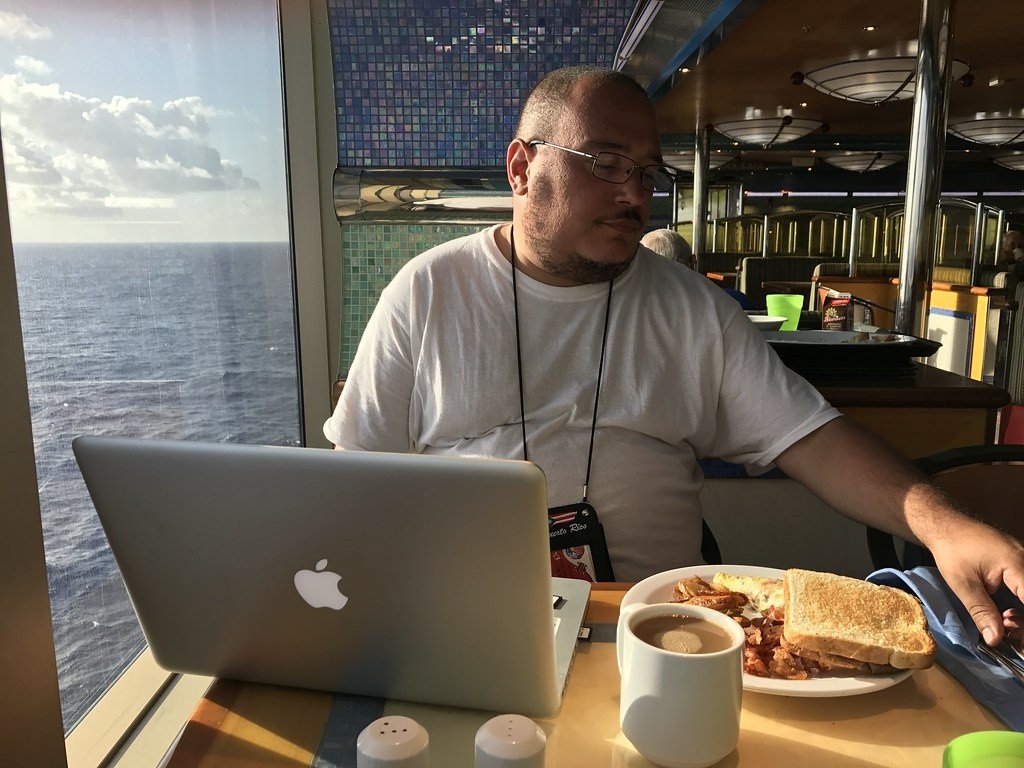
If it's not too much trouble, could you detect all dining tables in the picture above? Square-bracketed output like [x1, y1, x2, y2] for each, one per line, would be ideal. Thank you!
[151, 577, 1009, 768]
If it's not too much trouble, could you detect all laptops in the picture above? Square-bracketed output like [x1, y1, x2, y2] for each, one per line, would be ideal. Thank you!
[72, 435, 592, 721]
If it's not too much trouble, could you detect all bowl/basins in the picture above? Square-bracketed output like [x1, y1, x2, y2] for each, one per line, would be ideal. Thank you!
[747, 315, 789, 331]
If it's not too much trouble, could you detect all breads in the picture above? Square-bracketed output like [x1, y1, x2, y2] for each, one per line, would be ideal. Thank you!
[783, 567, 936, 674]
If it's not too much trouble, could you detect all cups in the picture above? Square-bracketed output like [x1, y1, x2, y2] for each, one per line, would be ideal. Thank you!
[615, 601, 745, 768]
[941, 730, 1024, 768]
[766, 294, 804, 331]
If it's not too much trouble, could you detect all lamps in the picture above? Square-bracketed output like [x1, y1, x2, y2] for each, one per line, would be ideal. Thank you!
[704, 116, 831, 151]
[992, 153, 1024, 172]
[791, 56, 976, 109]
[945, 118, 1024, 147]
[815, 151, 910, 176]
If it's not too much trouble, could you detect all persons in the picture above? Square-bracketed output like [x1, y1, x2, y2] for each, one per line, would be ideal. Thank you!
[640, 229, 748, 306]
[323, 66, 1022, 646]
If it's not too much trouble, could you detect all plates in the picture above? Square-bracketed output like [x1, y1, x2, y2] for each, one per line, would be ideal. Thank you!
[620, 564, 916, 698]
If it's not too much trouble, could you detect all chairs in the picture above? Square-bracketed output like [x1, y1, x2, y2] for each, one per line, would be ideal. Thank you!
[865, 440, 1024, 570]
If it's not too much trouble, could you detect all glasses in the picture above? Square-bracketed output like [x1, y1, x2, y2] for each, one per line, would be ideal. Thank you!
[528, 138, 680, 195]
